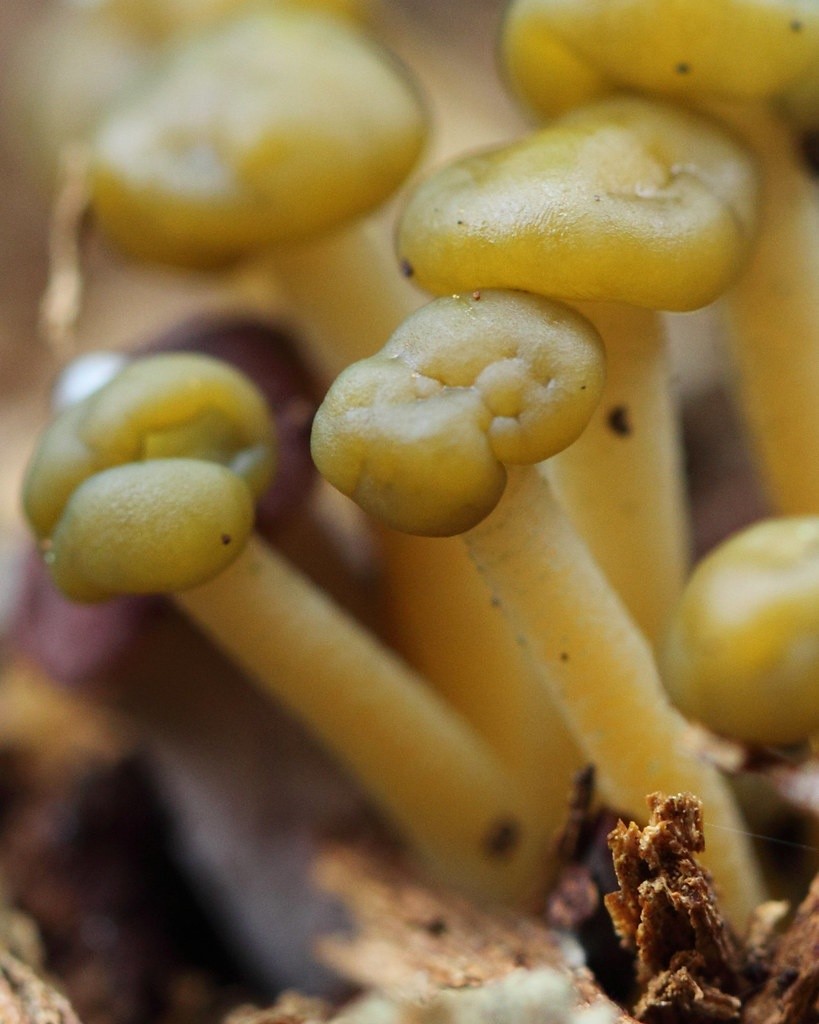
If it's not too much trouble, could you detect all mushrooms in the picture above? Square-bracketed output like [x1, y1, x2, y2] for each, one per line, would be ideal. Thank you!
[1, 0, 818, 946]
